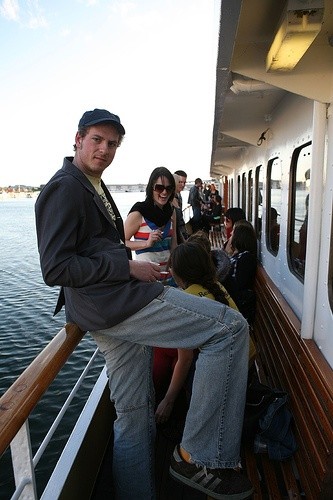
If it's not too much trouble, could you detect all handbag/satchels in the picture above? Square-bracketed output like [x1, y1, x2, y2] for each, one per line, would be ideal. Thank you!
[244, 386, 295, 455]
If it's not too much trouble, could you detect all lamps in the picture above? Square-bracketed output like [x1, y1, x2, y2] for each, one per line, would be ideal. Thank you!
[265, 0, 324, 72]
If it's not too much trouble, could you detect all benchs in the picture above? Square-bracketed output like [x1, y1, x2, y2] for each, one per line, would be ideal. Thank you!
[207, 224, 333, 500]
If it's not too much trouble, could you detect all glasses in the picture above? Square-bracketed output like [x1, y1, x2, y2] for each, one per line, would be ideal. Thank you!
[153, 184, 174, 194]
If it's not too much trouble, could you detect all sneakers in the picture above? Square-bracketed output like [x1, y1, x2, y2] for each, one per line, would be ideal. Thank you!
[169, 446, 254, 499]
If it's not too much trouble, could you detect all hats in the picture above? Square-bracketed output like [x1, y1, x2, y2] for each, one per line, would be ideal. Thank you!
[78, 108, 126, 135]
